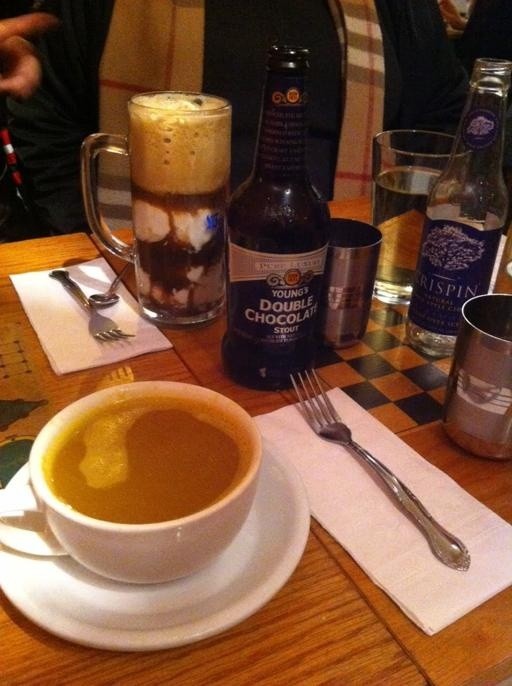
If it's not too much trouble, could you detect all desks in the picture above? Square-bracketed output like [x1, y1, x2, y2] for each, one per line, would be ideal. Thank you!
[0, 181, 511, 686]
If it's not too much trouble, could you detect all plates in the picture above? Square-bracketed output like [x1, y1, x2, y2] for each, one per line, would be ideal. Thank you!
[0, 433, 312, 655]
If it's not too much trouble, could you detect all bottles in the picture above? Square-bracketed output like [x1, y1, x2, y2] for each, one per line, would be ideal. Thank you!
[407, 56, 511, 362]
[222, 41, 333, 393]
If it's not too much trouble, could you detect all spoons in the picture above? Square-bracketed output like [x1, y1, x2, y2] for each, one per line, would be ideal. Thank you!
[88, 262, 136, 306]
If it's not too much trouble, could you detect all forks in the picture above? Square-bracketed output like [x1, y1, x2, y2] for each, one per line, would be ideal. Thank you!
[289, 367, 472, 575]
[50, 268, 136, 343]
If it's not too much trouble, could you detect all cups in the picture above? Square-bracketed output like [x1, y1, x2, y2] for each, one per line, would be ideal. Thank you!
[326, 214, 384, 351]
[79, 89, 231, 330]
[444, 294, 512, 463]
[0, 377, 265, 587]
[366, 127, 473, 307]
